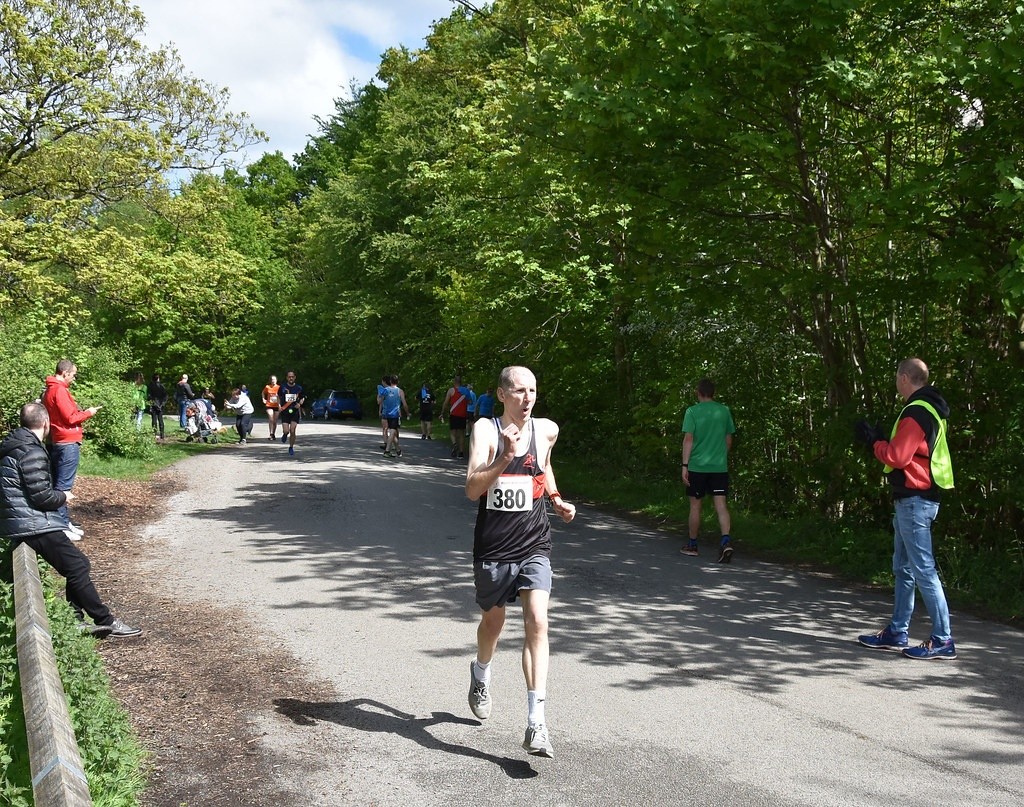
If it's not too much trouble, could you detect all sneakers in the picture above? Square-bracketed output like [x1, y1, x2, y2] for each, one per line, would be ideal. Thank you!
[109, 618, 143, 637]
[679, 543, 698, 556]
[522, 723, 553, 758]
[718, 542, 733, 563]
[467, 657, 493, 719]
[858, 624, 909, 650]
[77, 620, 113, 639]
[902, 636, 957, 660]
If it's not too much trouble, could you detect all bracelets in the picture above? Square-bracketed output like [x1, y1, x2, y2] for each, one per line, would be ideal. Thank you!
[547, 493, 562, 507]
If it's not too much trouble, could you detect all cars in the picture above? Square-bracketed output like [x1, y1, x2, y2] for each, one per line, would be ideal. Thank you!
[308, 388, 363, 420]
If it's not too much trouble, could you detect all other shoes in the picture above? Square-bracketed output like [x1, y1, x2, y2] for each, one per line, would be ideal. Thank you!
[390, 450, 403, 458]
[63, 491, 75, 500]
[70, 520, 82, 527]
[288, 447, 294, 455]
[427, 436, 431, 440]
[281, 434, 287, 443]
[382, 451, 390, 458]
[236, 439, 246, 446]
[457, 452, 464, 460]
[63, 522, 84, 541]
[422, 434, 426, 440]
[380, 443, 387, 450]
[269, 433, 276, 441]
[450, 443, 458, 457]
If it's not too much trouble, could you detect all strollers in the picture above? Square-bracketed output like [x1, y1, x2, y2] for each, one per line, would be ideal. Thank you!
[185, 399, 218, 443]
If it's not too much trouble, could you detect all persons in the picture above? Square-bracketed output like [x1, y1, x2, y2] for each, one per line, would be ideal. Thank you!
[415, 381, 436, 440]
[466, 365, 576, 758]
[44, 359, 97, 542]
[277, 370, 306, 455]
[680, 379, 736, 563]
[439, 376, 494, 459]
[202, 387, 215, 404]
[40, 390, 54, 456]
[225, 385, 254, 444]
[262, 375, 281, 441]
[133, 371, 167, 441]
[376, 374, 410, 457]
[177, 374, 195, 428]
[0, 402, 143, 638]
[855, 357, 959, 659]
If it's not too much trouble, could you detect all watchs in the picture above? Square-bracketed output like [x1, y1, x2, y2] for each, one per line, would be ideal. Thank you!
[680, 463, 688, 467]
